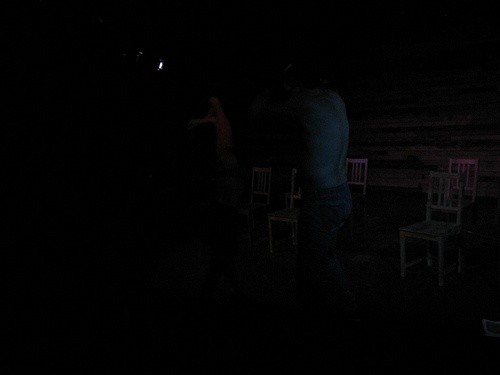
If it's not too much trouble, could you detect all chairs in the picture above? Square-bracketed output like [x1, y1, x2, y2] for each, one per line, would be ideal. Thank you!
[344, 158, 368, 239]
[266, 168, 299, 257]
[397, 171, 467, 287]
[436, 157, 480, 238]
[223, 162, 272, 252]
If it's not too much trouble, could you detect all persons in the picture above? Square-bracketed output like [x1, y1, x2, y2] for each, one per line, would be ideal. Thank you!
[276, 63, 369, 330]
[175, 90, 233, 167]
